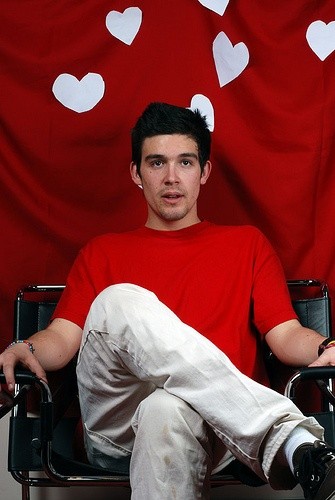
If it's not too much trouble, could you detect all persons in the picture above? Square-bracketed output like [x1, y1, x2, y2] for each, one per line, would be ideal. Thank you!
[0, 100, 335, 500]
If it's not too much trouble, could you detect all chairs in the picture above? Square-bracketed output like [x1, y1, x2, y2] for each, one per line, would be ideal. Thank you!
[0, 279, 335, 500]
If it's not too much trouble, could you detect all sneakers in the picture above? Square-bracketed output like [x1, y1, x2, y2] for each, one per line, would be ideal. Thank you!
[292, 438, 335, 500]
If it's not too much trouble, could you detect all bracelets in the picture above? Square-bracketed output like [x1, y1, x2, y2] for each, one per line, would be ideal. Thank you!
[318, 337, 335, 358]
[5, 339, 35, 354]
